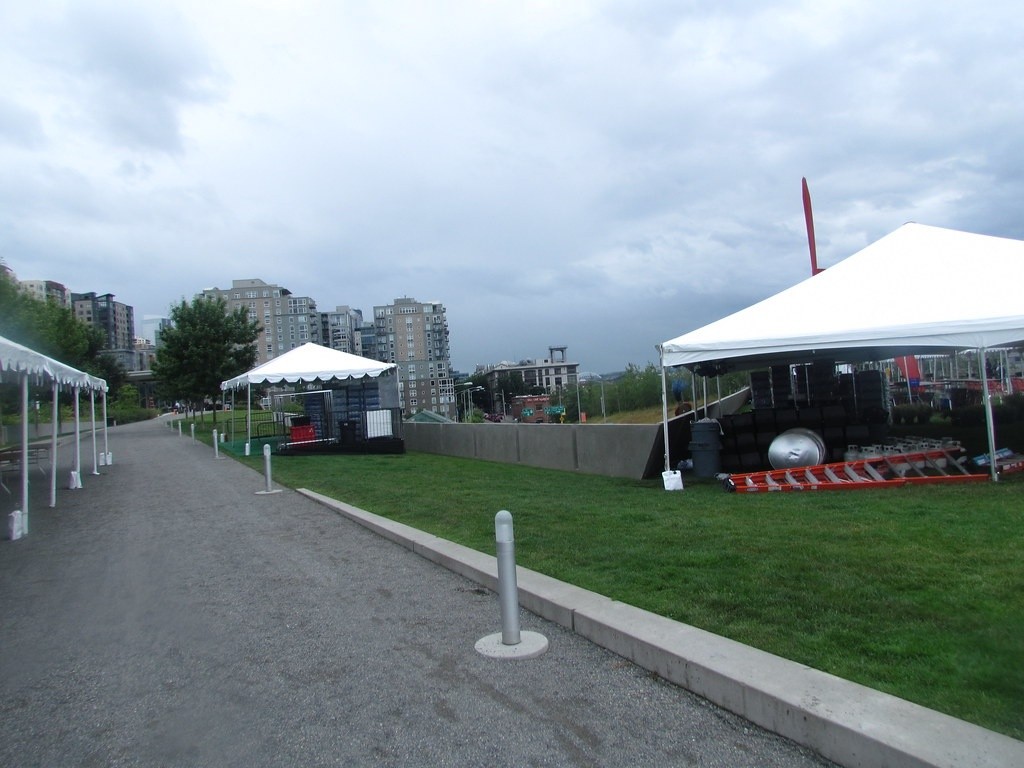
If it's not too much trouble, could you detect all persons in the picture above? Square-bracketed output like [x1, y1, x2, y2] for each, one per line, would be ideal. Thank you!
[862, 397, 889, 442]
[167, 394, 235, 414]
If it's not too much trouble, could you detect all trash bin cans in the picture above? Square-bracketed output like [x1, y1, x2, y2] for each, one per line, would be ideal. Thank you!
[338, 420, 356, 441]
[581, 412, 586, 422]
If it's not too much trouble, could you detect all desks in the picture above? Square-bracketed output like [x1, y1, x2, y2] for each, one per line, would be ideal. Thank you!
[0, 442, 61, 494]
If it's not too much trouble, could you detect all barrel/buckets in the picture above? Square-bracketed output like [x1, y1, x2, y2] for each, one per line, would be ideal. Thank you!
[661, 468, 684, 491]
[688, 358, 891, 479]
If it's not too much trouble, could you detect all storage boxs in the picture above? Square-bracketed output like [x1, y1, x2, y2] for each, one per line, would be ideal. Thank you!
[955, 438, 1024, 474]
[718, 360, 890, 472]
[290, 416, 317, 448]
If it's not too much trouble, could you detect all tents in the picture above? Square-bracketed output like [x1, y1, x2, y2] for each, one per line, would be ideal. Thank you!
[654, 220, 1024, 491]
[221, 341, 404, 456]
[0, 336, 113, 542]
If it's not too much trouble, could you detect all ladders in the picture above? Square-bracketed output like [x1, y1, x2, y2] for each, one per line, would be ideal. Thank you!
[722, 446, 991, 494]
